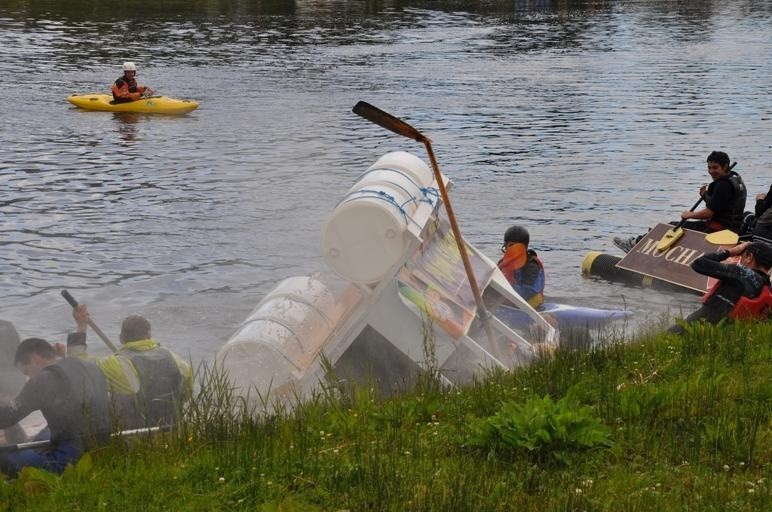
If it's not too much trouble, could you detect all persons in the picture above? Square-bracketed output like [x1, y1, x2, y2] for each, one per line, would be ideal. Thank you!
[482, 226, 545, 329]
[66, 305, 195, 447]
[739, 184, 772, 238]
[663, 238, 772, 338]
[112, 61, 154, 104]
[613, 151, 747, 254]
[0, 339, 113, 474]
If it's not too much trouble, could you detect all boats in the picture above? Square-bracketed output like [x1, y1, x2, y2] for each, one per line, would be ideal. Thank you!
[580, 251, 700, 289]
[66, 92, 200, 116]
[482, 294, 634, 327]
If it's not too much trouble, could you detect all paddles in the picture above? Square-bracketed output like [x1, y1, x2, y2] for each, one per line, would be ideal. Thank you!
[705, 230, 755, 246]
[657, 161, 740, 251]
[352, 100, 503, 365]
[1, 411, 188, 455]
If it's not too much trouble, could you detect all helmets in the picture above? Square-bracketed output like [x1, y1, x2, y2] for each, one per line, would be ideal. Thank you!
[123, 62, 136, 71]
[503, 226, 529, 243]
